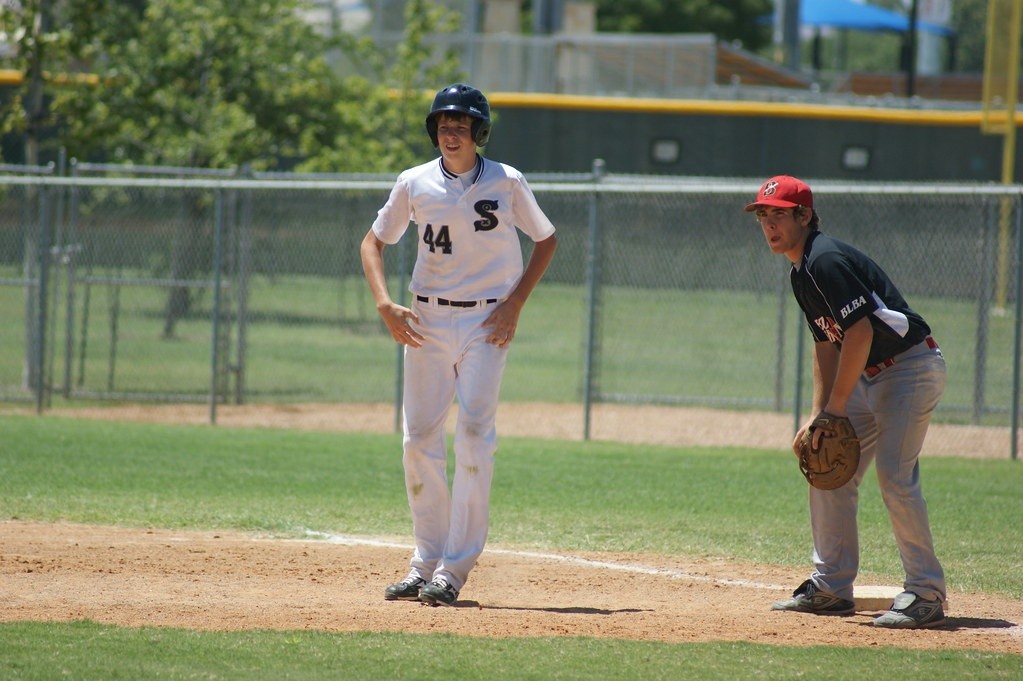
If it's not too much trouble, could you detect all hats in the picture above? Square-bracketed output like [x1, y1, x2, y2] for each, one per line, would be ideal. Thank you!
[744, 174, 814, 213]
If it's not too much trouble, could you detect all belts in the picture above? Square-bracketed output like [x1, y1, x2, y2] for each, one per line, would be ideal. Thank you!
[417, 295, 497, 307]
[864, 336, 940, 379]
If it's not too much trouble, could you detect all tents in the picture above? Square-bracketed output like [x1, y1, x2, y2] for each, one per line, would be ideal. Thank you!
[756, 0, 957, 74]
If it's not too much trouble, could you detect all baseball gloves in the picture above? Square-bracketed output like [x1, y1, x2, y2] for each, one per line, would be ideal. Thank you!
[798, 410, 861, 491]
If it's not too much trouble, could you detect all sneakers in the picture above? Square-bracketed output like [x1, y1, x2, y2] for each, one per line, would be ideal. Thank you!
[418, 576, 459, 606]
[384, 576, 427, 601]
[770, 577, 855, 616]
[874, 589, 946, 629]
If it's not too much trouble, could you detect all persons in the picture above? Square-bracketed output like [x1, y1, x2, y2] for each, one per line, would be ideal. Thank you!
[743, 175, 948, 629]
[361, 85, 558, 605]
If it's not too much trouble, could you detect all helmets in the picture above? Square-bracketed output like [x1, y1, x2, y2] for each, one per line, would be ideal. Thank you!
[426, 82, 491, 148]
